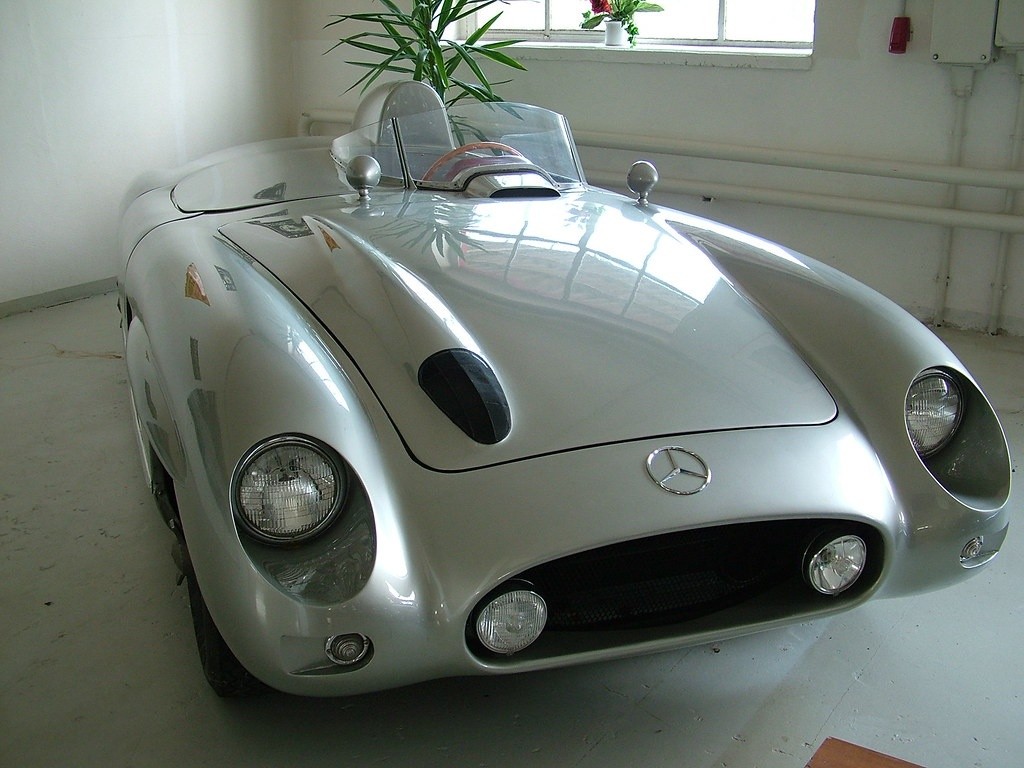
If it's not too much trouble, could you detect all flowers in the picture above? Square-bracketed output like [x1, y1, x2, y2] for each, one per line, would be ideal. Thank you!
[579, 0, 664, 47]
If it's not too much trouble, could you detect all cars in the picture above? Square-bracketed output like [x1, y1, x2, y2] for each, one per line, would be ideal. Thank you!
[114, 79, 1011, 719]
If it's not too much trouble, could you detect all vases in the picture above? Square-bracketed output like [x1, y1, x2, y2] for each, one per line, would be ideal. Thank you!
[605, 21, 630, 45]
